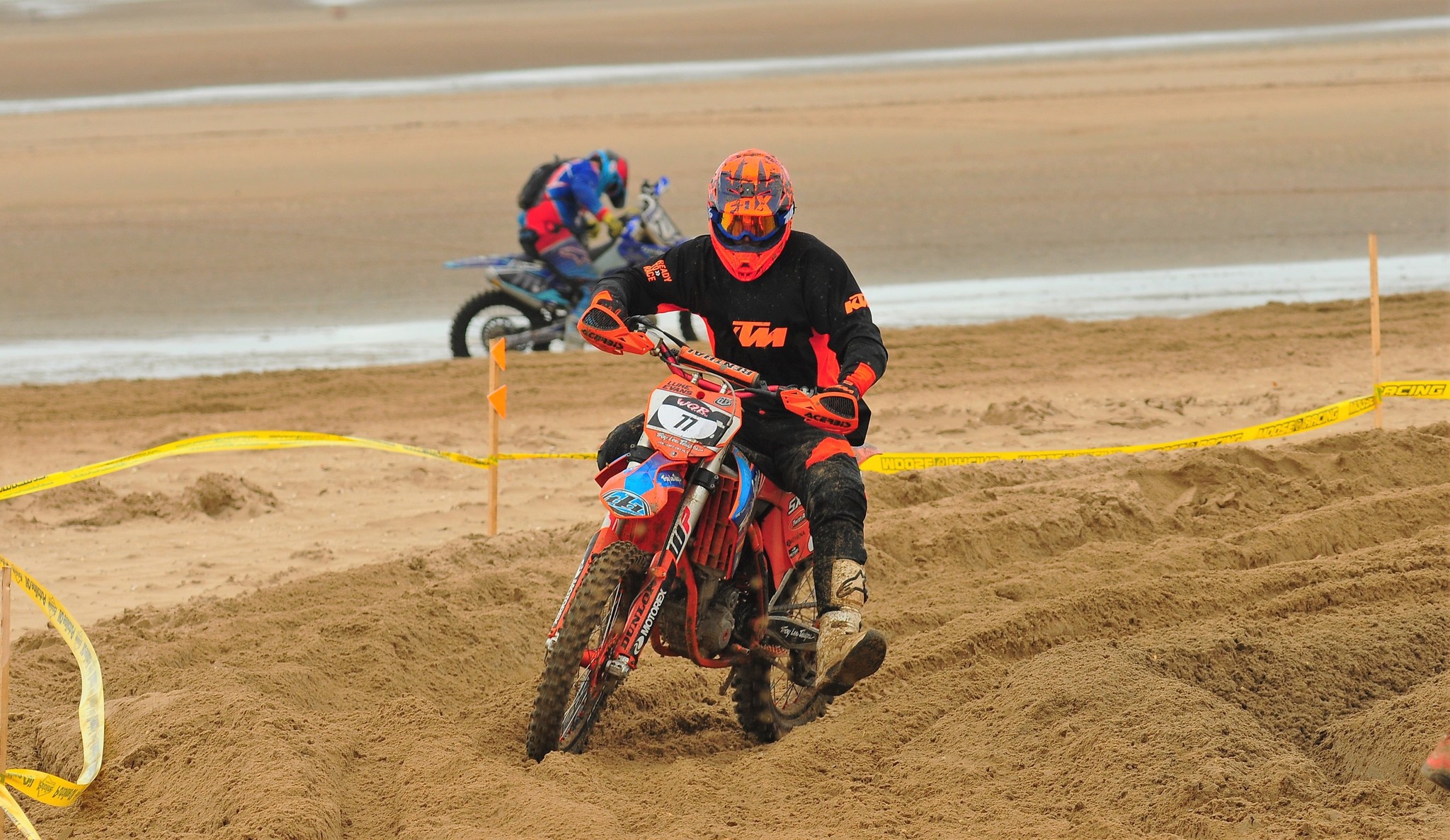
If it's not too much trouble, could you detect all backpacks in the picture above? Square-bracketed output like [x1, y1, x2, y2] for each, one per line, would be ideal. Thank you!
[519, 157, 575, 210]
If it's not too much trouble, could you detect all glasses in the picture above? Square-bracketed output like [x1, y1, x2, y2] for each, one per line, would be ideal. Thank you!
[711, 208, 786, 242]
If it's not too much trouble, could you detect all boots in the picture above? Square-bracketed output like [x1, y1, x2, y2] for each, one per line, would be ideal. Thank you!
[815, 611, 887, 696]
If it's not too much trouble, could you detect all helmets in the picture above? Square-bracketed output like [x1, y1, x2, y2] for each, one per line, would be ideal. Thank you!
[707, 149, 796, 281]
[586, 150, 628, 208]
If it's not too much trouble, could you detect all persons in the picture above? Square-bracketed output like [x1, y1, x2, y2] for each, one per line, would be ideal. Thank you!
[577, 149, 889, 695]
[518, 148, 628, 341]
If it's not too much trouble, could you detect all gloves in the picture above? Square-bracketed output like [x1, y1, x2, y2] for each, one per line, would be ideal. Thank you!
[587, 219, 600, 238]
[593, 293, 629, 328]
[603, 214, 623, 238]
[822, 379, 860, 415]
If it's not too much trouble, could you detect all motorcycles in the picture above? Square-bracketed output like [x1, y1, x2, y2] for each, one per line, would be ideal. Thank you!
[441, 175, 704, 357]
[521, 294, 864, 767]
